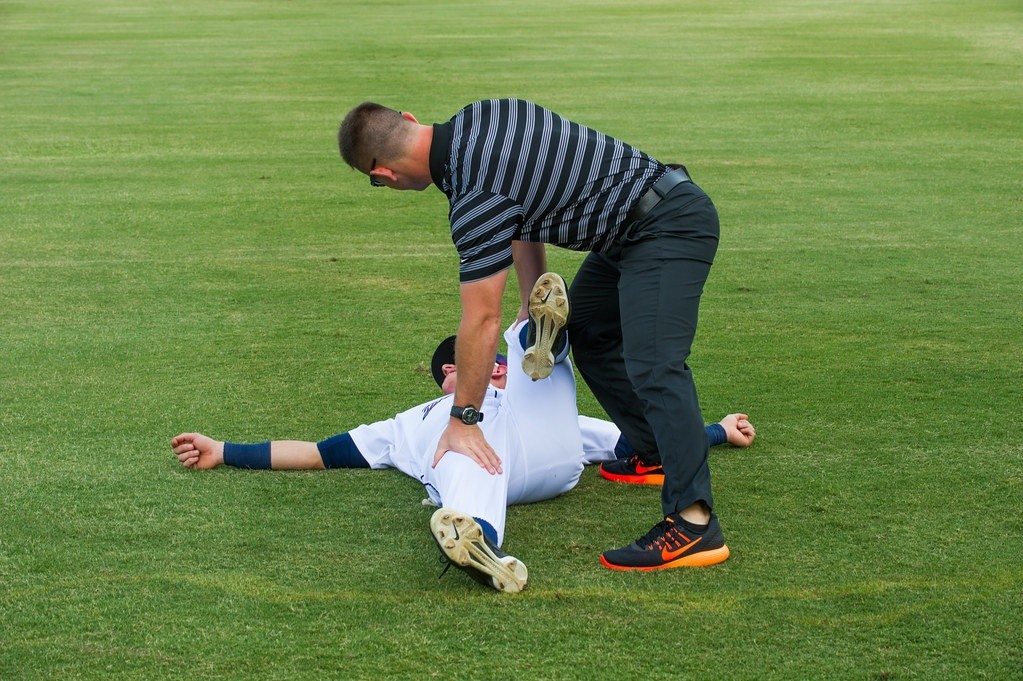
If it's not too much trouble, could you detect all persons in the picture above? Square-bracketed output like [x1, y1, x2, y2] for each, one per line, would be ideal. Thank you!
[336, 97, 729, 572]
[172, 272, 755, 593]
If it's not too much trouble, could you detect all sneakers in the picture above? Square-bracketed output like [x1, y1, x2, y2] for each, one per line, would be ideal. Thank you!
[600, 514, 728, 572]
[599, 454, 665, 485]
[521, 272, 571, 382]
[429, 508, 528, 594]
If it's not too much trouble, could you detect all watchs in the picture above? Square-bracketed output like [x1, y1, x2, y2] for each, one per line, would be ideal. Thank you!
[450, 405, 485, 425]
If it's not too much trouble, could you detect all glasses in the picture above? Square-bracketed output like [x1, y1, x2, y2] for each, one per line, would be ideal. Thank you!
[370, 111, 401, 187]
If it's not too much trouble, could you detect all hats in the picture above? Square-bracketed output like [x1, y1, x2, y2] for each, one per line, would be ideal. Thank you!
[431, 334, 507, 389]
